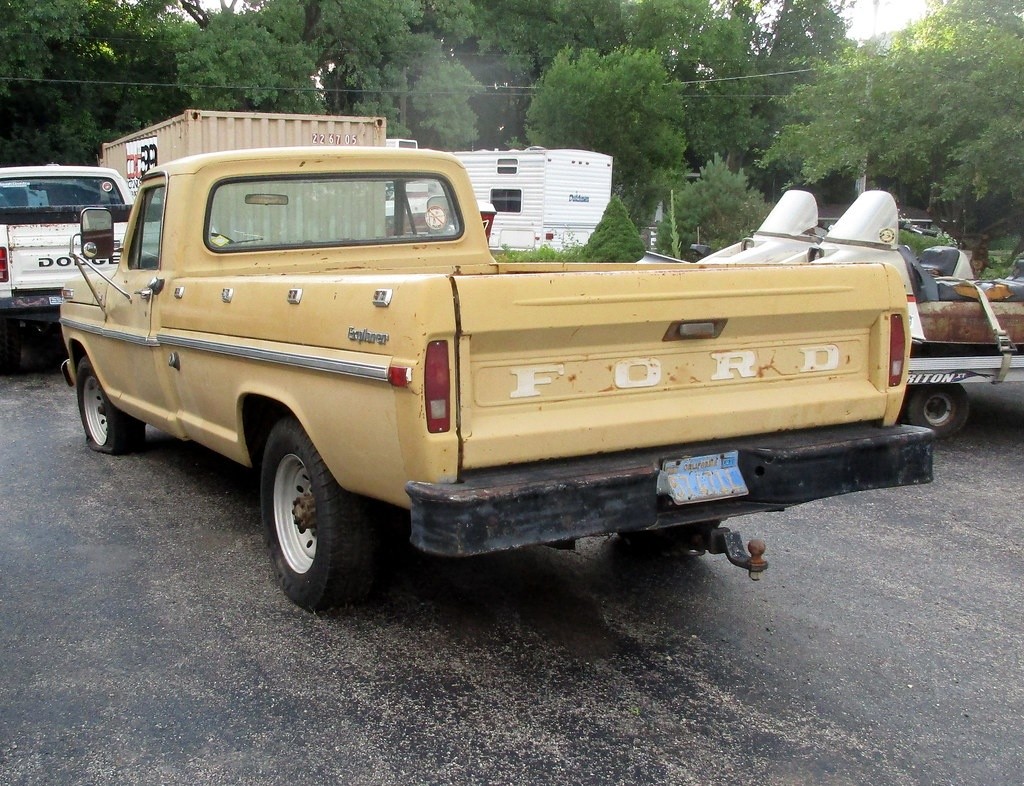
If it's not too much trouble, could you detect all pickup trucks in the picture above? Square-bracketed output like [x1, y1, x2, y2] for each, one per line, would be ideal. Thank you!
[58, 145, 935, 617]
[96, 109, 388, 246]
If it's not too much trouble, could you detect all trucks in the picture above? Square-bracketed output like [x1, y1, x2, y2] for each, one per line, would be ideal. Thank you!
[0, 163, 164, 376]
[384, 145, 614, 253]
